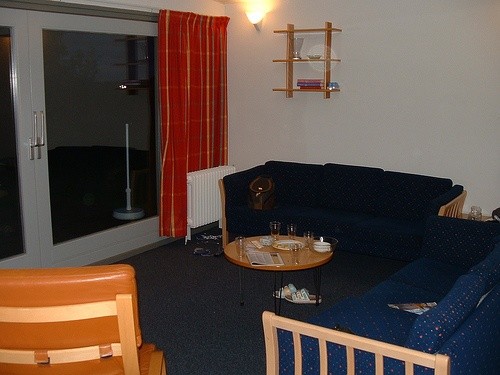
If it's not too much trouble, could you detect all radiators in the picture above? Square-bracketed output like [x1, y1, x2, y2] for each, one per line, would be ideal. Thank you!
[185, 163, 236, 245]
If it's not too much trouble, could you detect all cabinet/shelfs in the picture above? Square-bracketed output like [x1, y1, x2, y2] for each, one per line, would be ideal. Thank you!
[272, 22, 342, 98]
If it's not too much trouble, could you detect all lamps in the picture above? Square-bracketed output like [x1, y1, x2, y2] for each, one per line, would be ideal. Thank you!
[247, 11, 263, 31]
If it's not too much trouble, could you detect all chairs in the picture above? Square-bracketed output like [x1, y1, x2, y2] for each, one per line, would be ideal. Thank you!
[0, 264, 167, 375]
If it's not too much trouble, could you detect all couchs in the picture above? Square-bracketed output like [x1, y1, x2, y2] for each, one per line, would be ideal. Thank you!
[261, 215, 500, 375]
[218, 160, 467, 263]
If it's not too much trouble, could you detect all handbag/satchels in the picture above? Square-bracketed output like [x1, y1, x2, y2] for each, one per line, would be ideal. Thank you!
[249, 175, 274, 209]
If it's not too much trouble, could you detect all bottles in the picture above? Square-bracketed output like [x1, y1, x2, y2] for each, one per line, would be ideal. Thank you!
[468, 205, 482, 221]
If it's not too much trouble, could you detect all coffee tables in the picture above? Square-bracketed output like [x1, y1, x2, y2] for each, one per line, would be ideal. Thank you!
[223, 235, 333, 315]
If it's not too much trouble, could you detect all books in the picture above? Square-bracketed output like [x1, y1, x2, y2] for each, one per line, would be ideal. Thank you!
[296, 79, 325, 89]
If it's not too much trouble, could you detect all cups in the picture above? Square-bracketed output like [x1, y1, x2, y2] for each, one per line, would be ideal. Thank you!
[270, 221, 280, 240]
[290, 243, 302, 264]
[304, 230, 313, 249]
[235, 236, 246, 258]
[287, 224, 297, 240]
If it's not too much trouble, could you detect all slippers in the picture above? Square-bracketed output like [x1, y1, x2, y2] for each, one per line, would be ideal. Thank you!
[272, 284, 297, 298]
[285, 288, 321, 304]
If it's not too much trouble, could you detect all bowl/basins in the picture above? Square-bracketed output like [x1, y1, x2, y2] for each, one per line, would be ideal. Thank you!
[308, 236, 338, 256]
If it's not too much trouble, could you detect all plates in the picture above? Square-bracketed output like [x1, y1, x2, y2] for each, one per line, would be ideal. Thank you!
[271, 240, 303, 250]
